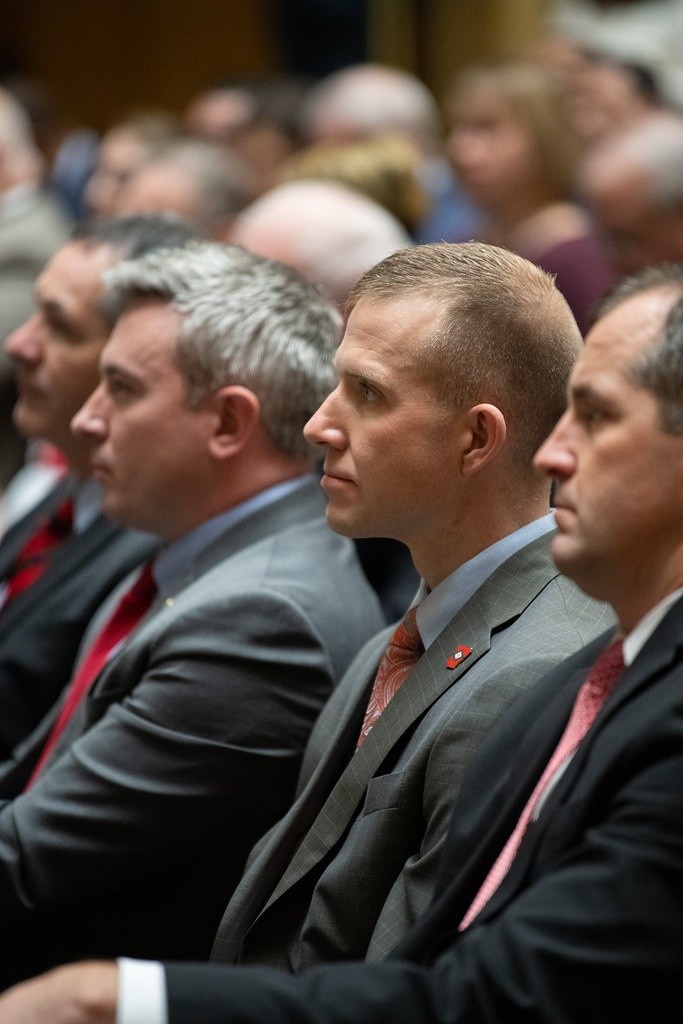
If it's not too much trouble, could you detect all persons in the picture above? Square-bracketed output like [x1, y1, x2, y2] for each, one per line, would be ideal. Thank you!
[5, 215, 203, 805]
[212, 237, 616, 1022]
[0, 7, 683, 628]
[0, 261, 683, 1022]
[5, 239, 386, 981]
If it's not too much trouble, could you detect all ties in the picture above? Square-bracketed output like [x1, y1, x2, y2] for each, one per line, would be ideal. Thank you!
[353, 605, 429, 759]
[22, 565, 159, 793]
[454, 641, 627, 930]
[6, 494, 73, 614]
